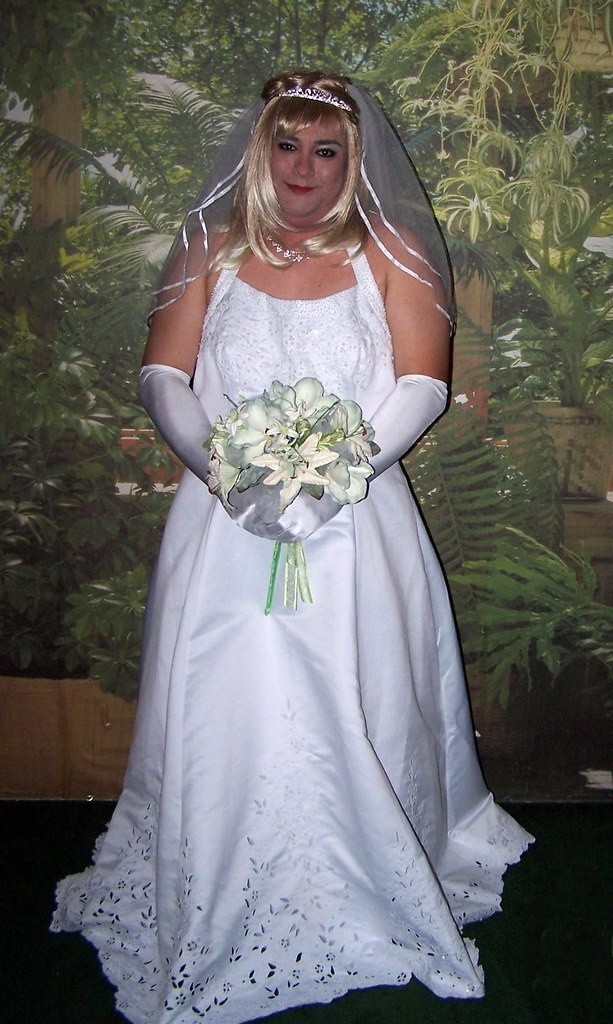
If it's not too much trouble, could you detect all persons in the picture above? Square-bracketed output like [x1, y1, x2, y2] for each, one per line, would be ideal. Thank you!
[52, 67, 536, 1024]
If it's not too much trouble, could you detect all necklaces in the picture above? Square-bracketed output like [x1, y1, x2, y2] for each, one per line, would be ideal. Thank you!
[267, 234, 310, 262]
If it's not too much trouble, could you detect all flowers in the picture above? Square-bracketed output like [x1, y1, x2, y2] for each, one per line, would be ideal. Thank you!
[201, 377, 382, 514]
